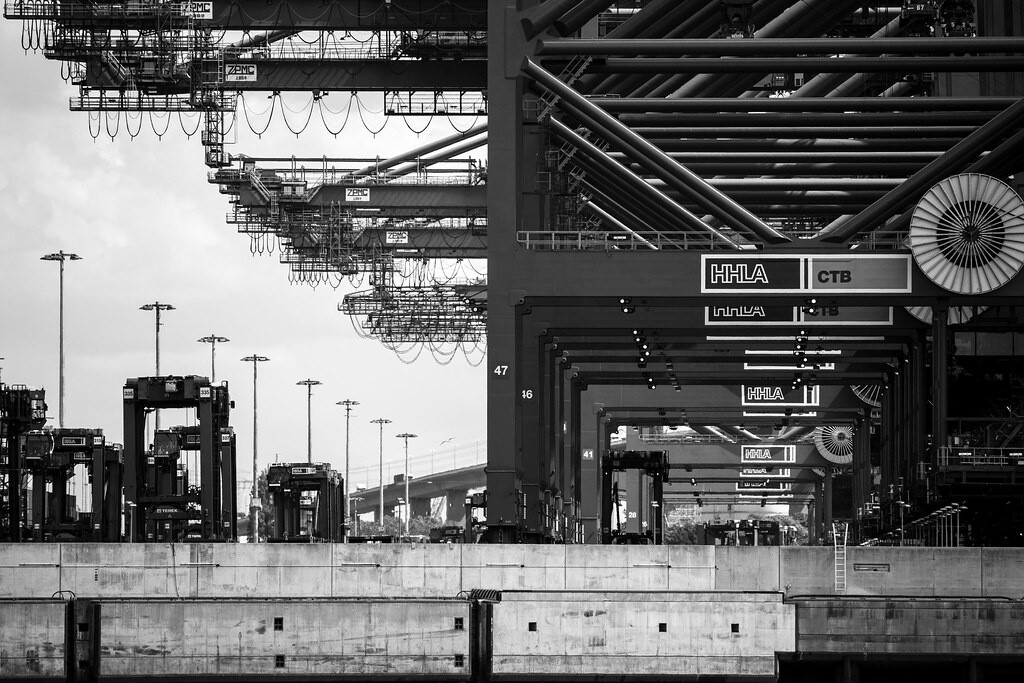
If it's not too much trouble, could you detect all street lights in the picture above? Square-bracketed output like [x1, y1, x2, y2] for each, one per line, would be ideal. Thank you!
[913, 503, 959, 548]
[396, 433, 418, 534]
[370, 417, 391, 535]
[240, 354, 271, 542]
[139, 301, 176, 377]
[897, 500, 910, 546]
[652, 501, 660, 545]
[197, 334, 229, 382]
[295, 377, 324, 465]
[336, 399, 360, 542]
[41, 250, 83, 427]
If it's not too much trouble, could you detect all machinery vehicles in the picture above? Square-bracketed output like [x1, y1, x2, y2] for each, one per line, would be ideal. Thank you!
[822, 520, 850, 546]
